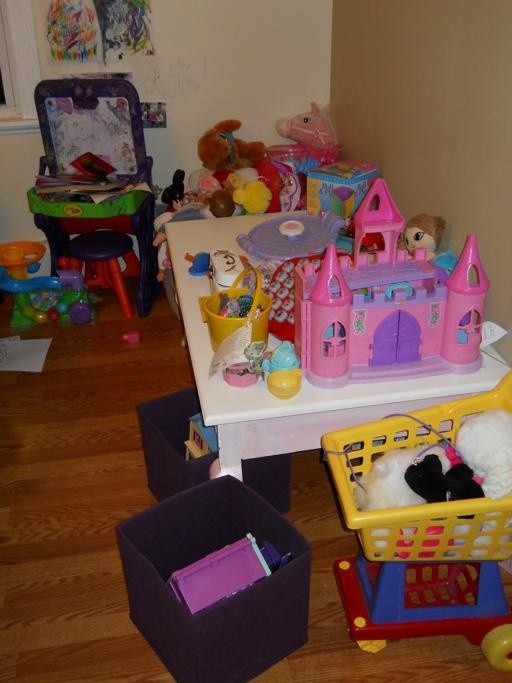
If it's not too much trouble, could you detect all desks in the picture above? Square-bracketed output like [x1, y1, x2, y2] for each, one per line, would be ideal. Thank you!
[163, 211, 510, 482]
[29, 77, 159, 317]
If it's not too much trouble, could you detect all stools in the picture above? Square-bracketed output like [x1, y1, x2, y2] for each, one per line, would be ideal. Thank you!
[68, 232, 141, 322]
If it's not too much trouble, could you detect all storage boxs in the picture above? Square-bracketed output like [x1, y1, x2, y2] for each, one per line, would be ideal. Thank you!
[134, 387, 294, 511]
[117, 474, 314, 681]
